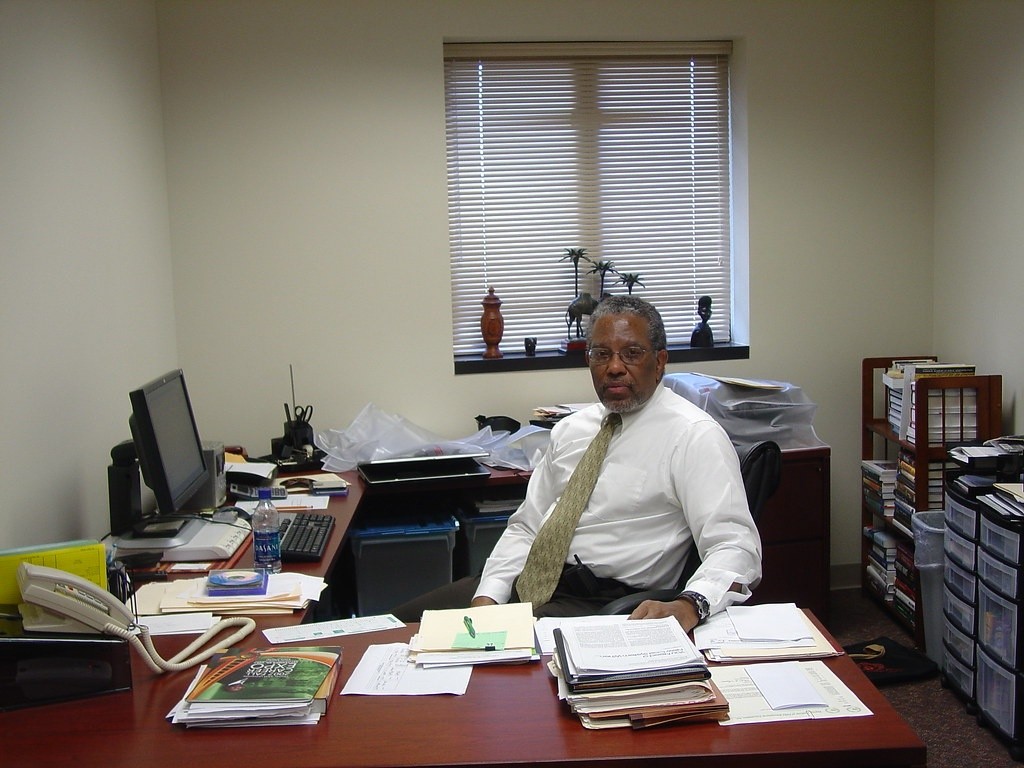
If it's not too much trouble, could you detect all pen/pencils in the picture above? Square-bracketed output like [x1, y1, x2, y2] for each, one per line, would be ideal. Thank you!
[463, 617, 475, 638]
[252, 505, 314, 508]
[284, 403, 291, 422]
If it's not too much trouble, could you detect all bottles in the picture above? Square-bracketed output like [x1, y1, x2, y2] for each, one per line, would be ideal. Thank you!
[252, 488, 281, 574]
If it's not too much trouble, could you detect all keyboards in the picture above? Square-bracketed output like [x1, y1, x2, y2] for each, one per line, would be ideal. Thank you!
[278, 512, 336, 562]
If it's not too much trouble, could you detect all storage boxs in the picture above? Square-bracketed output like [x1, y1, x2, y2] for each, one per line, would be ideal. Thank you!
[349, 501, 459, 623]
[453, 499, 509, 574]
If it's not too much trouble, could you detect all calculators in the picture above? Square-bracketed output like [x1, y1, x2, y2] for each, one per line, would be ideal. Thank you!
[229, 482, 288, 501]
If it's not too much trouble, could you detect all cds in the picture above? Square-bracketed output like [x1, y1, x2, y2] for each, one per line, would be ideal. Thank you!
[210, 571, 262, 585]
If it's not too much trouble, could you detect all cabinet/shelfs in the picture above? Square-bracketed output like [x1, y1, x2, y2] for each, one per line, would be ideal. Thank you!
[938, 442, 1024, 759]
[726, 434, 834, 630]
[858, 355, 1004, 645]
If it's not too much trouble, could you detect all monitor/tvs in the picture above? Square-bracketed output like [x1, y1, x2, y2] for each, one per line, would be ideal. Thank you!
[106, 368, 210, 548]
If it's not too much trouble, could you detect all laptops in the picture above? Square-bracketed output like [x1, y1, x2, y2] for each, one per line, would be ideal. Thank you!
[356, 452, 492, 487]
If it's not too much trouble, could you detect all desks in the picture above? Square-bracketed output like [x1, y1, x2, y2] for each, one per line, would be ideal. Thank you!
[0, 445, 921, 768]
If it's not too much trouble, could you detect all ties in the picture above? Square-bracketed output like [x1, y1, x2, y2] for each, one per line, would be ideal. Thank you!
[515, 413, 622, 610]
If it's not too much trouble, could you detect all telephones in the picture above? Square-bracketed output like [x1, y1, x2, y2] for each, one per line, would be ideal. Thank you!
[14, 558, 135, 634]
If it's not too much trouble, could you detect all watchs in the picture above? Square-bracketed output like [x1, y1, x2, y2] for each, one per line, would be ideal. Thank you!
[676, 591, 711, 623]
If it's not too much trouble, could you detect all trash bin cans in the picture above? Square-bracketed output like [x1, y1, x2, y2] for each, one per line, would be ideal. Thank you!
[912, 509, 945, 678]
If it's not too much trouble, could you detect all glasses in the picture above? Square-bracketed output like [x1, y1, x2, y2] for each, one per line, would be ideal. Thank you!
[585, 345, 656, 365]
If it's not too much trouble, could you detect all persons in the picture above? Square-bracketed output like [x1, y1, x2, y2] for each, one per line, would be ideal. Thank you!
[470, 295, 763, 634]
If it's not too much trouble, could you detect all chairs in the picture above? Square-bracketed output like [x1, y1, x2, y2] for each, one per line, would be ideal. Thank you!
[596, 437, 785, 614]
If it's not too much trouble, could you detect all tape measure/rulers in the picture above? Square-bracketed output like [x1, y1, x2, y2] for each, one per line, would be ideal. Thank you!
[289, 364, 302, 422]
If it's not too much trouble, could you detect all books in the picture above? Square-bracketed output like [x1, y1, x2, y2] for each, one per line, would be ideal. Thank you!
[861, 447, 965, 626]
[184, 645, 344, 717]
[554, 616, 730, 730]
[882, 358, 977, 443]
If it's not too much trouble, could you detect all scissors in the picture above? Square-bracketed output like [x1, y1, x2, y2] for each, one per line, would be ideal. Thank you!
[295, 405, 313, 423]
